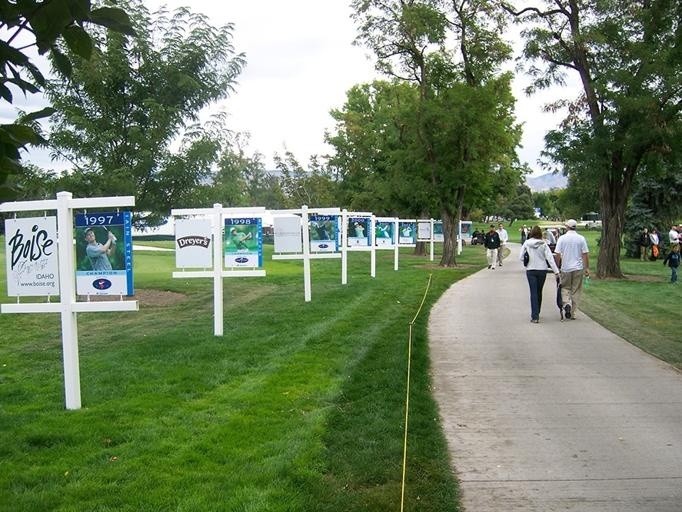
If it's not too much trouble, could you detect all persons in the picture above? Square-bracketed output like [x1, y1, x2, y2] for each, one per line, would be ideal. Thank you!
[517, 218, 590, 324]
[379, 225, 390, 237]
[230, 227, 253, 254]
[83, 227, 117, 270]
[471, 223, 508, 270]
[354, 222, 365, 237]
[312, 219, 331, 240]
[402, 226, 412, 236]
[638, 224, 682, 286]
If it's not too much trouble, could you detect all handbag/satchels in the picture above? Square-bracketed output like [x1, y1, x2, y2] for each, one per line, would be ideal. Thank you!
[523, 252, 529, 266]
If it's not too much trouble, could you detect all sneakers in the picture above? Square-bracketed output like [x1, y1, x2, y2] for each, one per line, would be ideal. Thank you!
[530, 319, 539, 323]
[564, 303, 576, 320]
[487, 262, 503, 269]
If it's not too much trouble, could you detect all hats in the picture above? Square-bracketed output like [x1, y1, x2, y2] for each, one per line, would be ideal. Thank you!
[354, 222, 359, 226]
[84, 228, 93, 238]
[231, 227, 235, 234]
[490, 224, 495, 228]
[563, 219, 577, 229]
